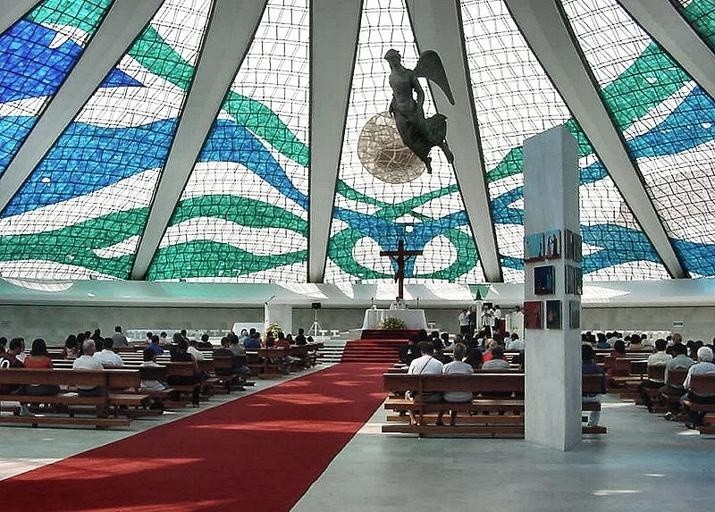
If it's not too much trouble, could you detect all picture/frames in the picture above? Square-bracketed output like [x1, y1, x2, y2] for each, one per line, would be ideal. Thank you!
[523, 227, 584, 330]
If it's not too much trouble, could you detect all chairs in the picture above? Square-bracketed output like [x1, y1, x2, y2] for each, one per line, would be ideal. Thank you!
[590, 342, 715, 435]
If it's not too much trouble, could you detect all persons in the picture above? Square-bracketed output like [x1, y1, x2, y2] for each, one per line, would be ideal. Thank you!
[1, 336, 62, 416]
[384, 48, 455, 173]
[61, 326, 128, 417]
[547, 235, 552, 255]
[398, 328, 523, 426]
[389, 296, 406, 309]
[457, 306, 523, 334]
[550, 234, 558, 255]
[138, 328, 311, 411]
[581, 330, 714, 427]
[390, 252, 413, 282]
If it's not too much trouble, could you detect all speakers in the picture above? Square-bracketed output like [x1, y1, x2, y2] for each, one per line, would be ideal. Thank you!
[484, 303, 492, 310]
[312, 303, 321, 309]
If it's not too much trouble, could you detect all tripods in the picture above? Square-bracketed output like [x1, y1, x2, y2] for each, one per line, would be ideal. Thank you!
[307, 309, 325, 337]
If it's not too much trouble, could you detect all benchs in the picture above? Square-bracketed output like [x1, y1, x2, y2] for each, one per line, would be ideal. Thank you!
[0, 340, 327, 430]
[379, 341, 607, 439]
